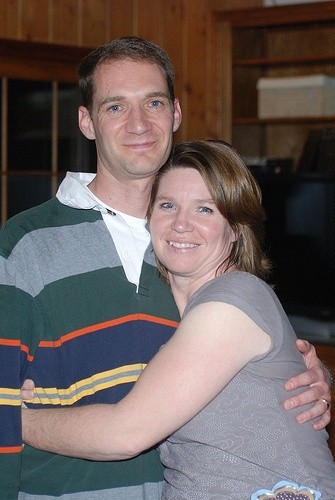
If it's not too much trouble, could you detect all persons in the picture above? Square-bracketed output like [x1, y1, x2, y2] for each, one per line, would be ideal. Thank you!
[20, 138, 335, 500]
[1, 35, 331, 500]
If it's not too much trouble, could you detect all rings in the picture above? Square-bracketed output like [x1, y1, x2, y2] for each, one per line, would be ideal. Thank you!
[320, 397, 331, 412]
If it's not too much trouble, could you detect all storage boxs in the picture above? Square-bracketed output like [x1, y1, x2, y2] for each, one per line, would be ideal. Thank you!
[256, 74, 335, 118]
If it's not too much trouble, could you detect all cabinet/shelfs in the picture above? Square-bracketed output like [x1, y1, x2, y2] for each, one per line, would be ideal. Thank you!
[231, 0, 334, 197]
